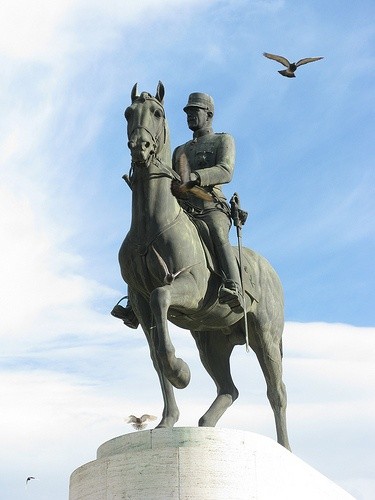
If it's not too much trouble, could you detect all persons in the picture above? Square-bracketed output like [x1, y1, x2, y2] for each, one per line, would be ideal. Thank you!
[112, 92, 245, 330]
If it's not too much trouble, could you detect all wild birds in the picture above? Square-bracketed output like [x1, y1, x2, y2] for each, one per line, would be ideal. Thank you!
[261, 52, 325, 78]
[26, 477, 35, 485]
[125, 414, 158, 430]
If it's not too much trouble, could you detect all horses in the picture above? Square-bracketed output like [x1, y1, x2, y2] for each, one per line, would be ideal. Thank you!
[118, 80, 291, 452]
